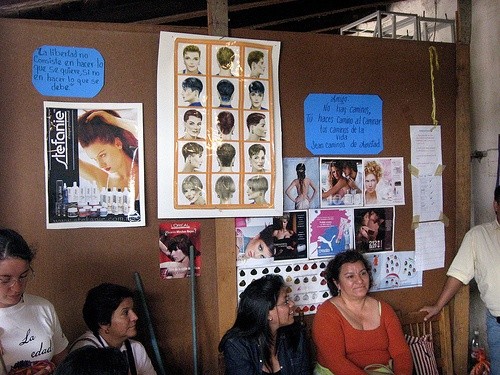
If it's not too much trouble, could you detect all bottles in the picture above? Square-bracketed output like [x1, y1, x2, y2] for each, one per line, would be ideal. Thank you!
[470, 330, 481, 366]
[298, 311, 307, 332]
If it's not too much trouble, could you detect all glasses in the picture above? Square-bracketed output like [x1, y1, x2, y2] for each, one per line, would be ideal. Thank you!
[275, 295, 290, 307]
[0, 264, 35, 288]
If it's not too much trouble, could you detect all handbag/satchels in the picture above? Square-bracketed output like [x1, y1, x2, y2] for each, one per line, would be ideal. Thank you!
[405, 332, 439, 375]
[365, 364, 394, 375]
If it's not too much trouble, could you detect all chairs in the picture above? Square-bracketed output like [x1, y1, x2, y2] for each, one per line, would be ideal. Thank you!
[396, 305, 454, 375]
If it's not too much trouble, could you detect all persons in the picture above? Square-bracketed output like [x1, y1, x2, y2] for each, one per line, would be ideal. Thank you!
[364, 161, 383, 204]
[272, 213, 298, 261]
[177, 42, 270, 205]
[284, 162, 318, 210]
[69, 282, 157, 375]
[77, 109, 142, 203]
[0, 229, 69, 375]
[322, 161, 363, 205]
[217, 273, 315, 375]
[358, 210, 386, 251]
[160, 234, 201, 279]
[417, 185, 500, 375]
[244, 224, 274, 259]
[310, 249, 414, 375]
